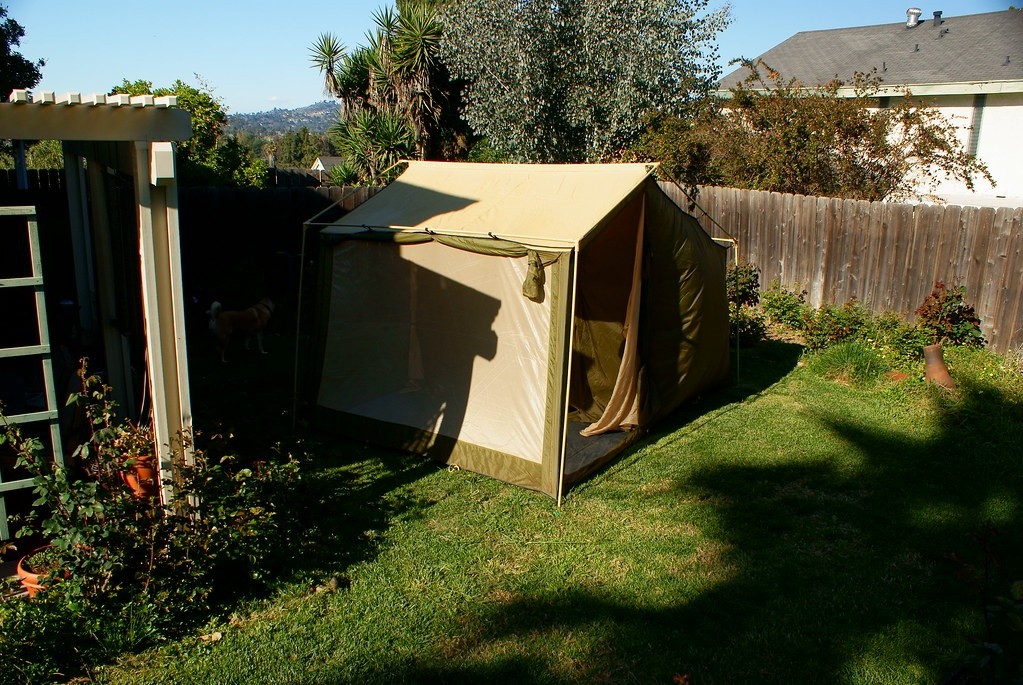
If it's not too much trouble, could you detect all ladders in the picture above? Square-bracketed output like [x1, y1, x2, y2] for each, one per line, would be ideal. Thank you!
[0, 195, 75, 565]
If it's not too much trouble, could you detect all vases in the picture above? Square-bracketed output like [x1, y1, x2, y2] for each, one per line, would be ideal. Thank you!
[17, 543, 94, 604]
[113, 455, 158, 497]
[923, 344, 956, 389]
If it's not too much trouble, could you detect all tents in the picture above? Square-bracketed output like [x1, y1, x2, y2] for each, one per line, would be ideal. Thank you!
[289, 159, 740, 512]
